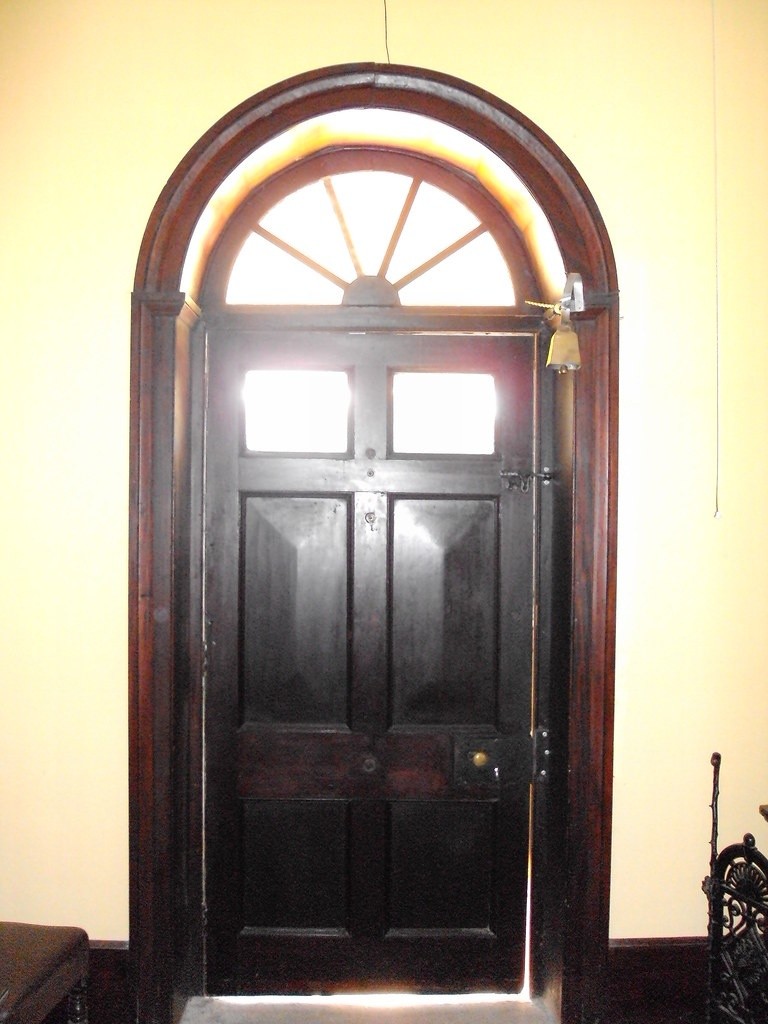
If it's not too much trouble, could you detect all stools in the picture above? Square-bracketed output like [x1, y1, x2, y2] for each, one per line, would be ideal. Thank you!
[0, 922, 90, 1024]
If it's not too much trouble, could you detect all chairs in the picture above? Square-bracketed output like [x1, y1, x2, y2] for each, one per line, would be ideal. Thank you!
[701, 832, 768, 1024]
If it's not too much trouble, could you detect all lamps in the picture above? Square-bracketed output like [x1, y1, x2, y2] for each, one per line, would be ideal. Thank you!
[545, 308, 583, 374]
